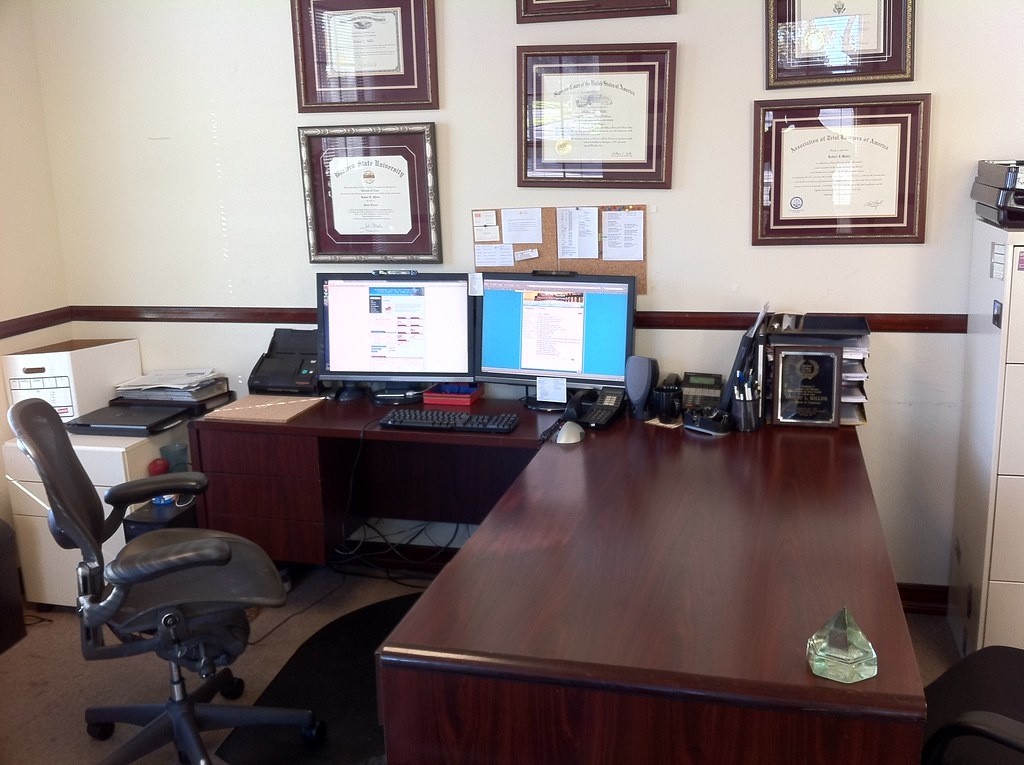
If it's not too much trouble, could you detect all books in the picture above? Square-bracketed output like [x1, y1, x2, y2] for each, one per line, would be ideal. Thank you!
[115, 368, 229, 402]
[718, 311, 764, 420]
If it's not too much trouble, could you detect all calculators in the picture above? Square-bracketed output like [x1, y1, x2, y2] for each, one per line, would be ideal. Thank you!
[680, 372, 722, 413]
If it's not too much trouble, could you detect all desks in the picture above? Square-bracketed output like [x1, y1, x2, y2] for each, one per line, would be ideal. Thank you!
[186, 392, 563, 582]
[370, 409, 931, 765]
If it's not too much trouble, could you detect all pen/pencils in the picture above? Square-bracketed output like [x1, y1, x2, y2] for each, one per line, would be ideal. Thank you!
[731, 368, 762, 433]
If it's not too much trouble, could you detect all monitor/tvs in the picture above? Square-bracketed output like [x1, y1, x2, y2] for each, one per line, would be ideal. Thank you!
[474, 271, 635, 413]
[316, 273, 475, 406]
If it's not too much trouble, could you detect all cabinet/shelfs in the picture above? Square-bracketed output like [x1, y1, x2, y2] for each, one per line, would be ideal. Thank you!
[942, 214, 1024, 654]
[3, 418, 192, 611]
[754, 305, 875, 433]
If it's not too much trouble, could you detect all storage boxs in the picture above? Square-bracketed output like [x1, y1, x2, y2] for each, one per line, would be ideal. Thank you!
[0, 334, 142, 424]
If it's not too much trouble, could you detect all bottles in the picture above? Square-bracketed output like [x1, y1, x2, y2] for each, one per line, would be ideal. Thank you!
[148, 442, 190, 504]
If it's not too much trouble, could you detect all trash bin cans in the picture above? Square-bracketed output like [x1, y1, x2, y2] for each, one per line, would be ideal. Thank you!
[0, 517, 28, 656]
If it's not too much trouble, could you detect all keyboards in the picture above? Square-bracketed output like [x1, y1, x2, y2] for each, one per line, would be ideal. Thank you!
[379, 408, 519, 433]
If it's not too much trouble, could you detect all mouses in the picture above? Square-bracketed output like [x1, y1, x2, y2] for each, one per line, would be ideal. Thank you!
[556, 421, 585, 443]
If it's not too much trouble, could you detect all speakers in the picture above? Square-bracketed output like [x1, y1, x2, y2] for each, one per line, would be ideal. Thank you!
[321, 377, 343, 400]
[623, 355, 660, 419]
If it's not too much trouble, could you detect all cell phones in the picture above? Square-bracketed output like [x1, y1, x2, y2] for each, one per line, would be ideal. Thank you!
[375, 389, 409, 397]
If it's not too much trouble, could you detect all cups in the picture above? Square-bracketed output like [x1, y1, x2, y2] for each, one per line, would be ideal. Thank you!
[652, 385, 684, 425]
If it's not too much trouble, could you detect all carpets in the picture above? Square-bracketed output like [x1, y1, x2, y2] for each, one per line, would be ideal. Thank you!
[214, 591, 424, 765]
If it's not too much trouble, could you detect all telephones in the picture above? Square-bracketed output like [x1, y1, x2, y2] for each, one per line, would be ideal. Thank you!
[558, 385, 625, 429]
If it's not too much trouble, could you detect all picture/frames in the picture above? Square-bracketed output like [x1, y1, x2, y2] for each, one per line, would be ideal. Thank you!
[761, 0, 920, 94]
[513, 37, 681, 191]
[749, 92, 932, 248]
[772, 342, 844, 429]
[296, 121, 448, 266]
[287, 0, 439, 114]
[514, 0, 679, 25]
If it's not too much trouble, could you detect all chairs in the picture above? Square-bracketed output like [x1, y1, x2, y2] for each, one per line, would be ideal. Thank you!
[922, 644, 1024, 765]
[14, 401, 327, 765]
